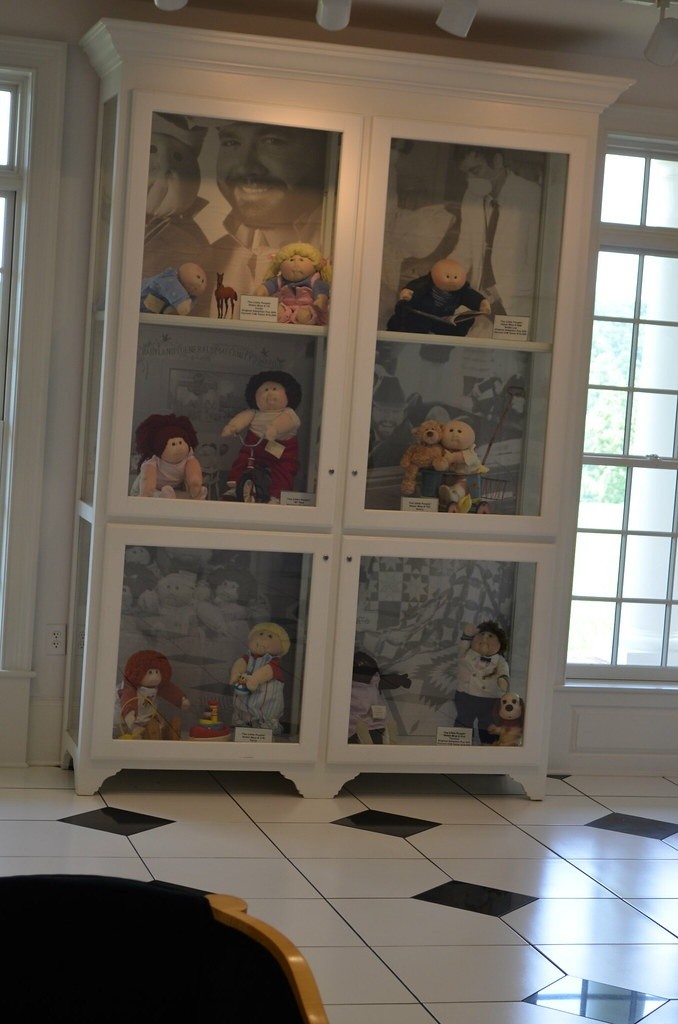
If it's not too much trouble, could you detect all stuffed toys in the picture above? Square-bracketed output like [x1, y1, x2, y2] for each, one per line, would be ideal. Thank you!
[399, 420, 449, 495]
[488, 693, 524, 746]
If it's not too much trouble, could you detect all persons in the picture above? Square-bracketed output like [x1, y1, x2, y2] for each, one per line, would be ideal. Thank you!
[253, 243, 333, 323]
[441, 145, 542, 315]
[140, 262, 208, 316]
[206, 118, 329, 319]
[386, 261, 491, 335]
[221, 371, 301, 504]
[122, 545, 269, 652]
[452, 622, 509, 746]
[142, 110, 217, 285]
[434, 423, 489, 502]
[367, 376, 419, 469]
[347, 653, 411, 743]
[129, 413, 209, 501]
[230, 622, 290, 734]
[119, 649, 192, 741]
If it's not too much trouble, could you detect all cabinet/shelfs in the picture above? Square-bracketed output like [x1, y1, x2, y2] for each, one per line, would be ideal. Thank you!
[60, 18, 636, 800]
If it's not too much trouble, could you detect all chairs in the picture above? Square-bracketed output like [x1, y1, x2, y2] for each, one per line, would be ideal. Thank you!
[0, 874, 329, 1024]
